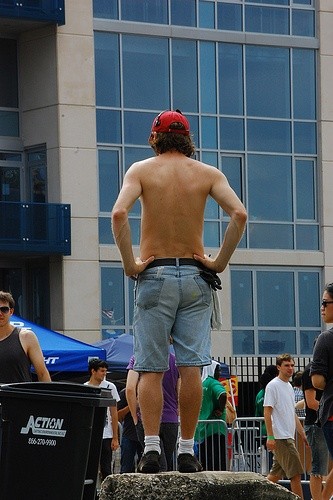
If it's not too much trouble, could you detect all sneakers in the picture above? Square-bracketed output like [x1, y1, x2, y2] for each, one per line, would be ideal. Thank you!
[137, 451, 160, 473]
[177, 453, 203, 473]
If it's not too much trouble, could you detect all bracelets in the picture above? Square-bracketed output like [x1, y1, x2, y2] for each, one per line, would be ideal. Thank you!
[113, 432, 118, 434]
[267, 436, 275, 440]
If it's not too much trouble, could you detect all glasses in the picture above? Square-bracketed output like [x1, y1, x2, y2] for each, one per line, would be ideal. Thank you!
[322, 301, 333, 307]
[0, 307, 9, 313]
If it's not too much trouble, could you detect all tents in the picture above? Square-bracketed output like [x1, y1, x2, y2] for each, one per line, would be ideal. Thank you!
[7, 312, 247, 473]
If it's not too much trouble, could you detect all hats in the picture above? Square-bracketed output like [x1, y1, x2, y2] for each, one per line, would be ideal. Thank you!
[152, 109, 190, 134]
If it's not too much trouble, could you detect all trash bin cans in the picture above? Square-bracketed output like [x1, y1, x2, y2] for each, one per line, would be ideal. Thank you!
[0, 381, 119, 500]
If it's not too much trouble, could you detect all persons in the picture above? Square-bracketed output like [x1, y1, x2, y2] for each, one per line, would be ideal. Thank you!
[309, 280, 333, 500]
[0, 290, 56, 383]
[111, 107, 249, 474]
[83, 344, 333, 500]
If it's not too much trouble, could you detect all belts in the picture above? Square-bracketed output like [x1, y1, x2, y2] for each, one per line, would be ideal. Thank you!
[144, 258, 209, 271]
